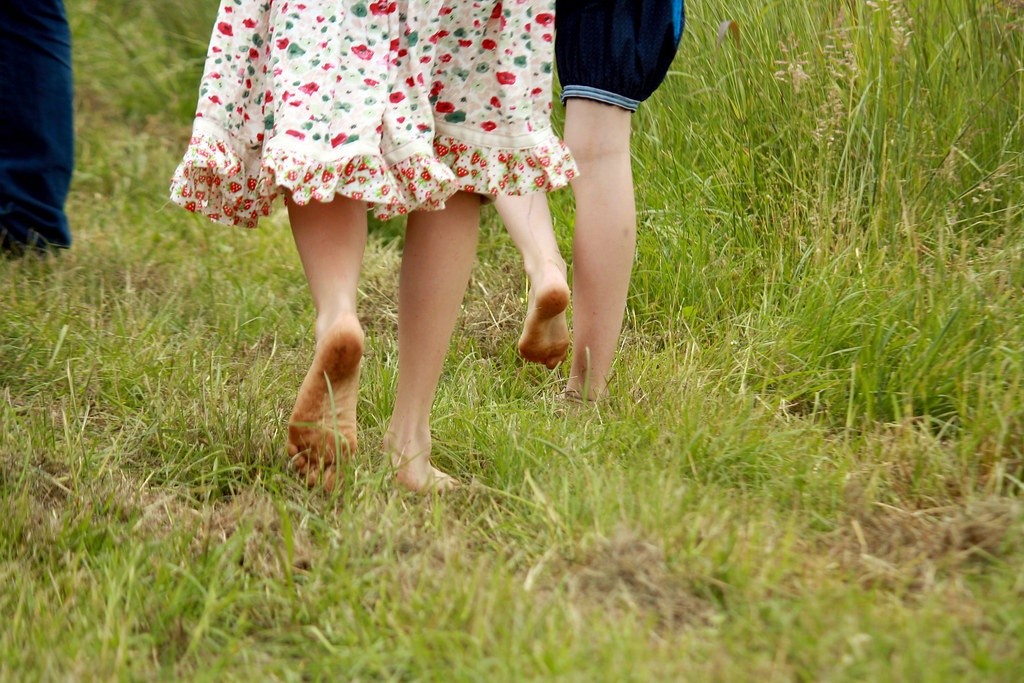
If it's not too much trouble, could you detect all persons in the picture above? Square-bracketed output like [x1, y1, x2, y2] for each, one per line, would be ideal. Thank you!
[490, 0, 686, 421]
[0, 0, 73, 260]
[166, 0, 580, 496]
